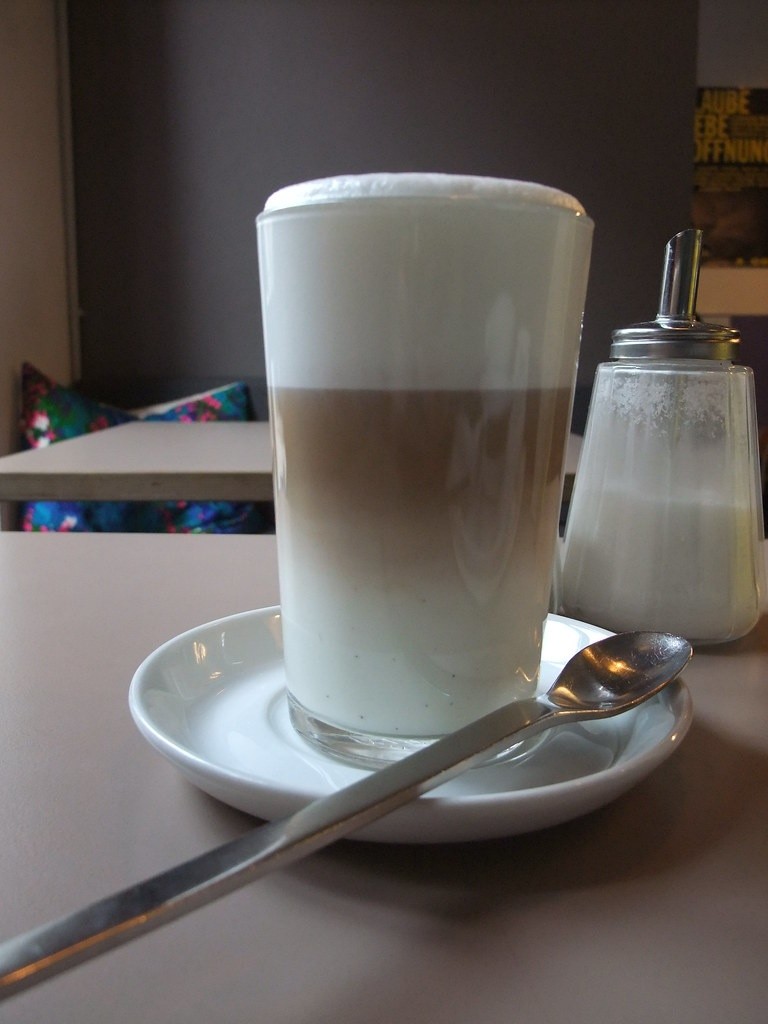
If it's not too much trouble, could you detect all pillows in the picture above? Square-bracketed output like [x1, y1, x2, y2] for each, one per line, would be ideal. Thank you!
[18, 361, 273, 532]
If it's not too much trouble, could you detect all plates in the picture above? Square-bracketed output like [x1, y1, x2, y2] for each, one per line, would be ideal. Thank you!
[128, 601, 688, 840]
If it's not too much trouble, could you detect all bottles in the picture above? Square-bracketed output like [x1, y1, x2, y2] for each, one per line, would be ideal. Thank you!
[560, 228, 762, 645]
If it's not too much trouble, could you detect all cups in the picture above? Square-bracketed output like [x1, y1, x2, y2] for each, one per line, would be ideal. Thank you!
[254, 196, 595, 768]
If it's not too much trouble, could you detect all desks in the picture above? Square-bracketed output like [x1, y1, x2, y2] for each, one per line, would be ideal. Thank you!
[0, 530, 767, 1023]
[0, 422, 583, 502]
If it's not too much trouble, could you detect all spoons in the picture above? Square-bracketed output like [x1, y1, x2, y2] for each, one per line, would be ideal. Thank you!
[1, 630, 693, 997]
